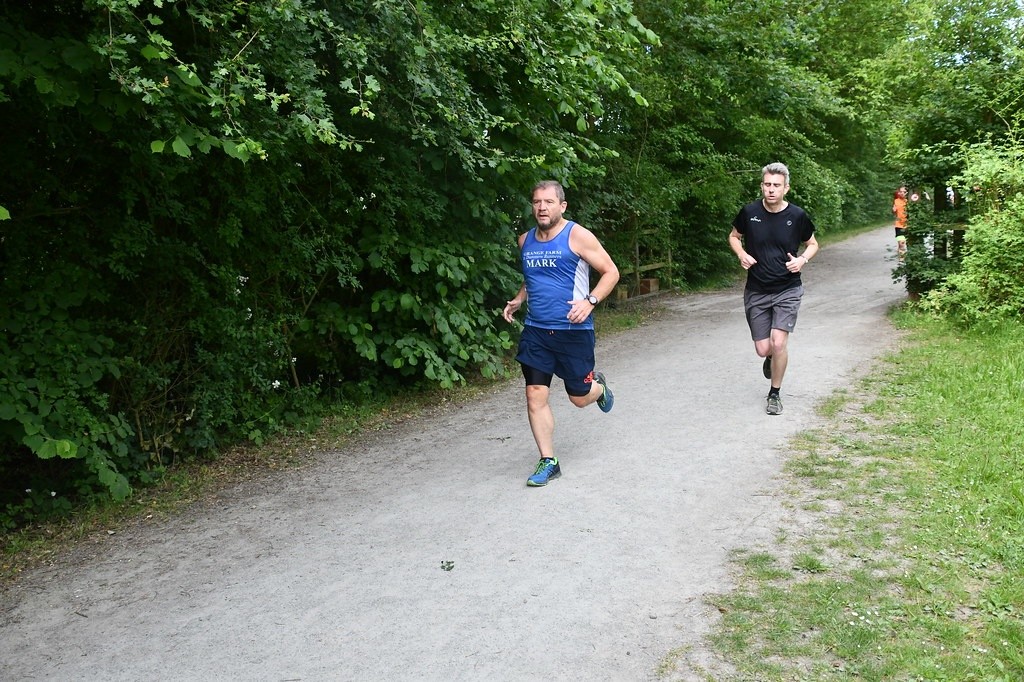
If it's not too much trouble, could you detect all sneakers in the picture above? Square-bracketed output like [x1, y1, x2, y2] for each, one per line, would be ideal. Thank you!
[592, 372, 614, 413]
[763, 355, 772, 379]
[527, 457, 562, 486]
[767, 392, 783, 415]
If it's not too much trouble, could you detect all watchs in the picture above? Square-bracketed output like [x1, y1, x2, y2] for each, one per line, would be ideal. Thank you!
[800, 254, 809, 265]
[585, 294, 598, 308]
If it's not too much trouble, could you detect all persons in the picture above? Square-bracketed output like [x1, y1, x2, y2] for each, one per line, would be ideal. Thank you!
[728, 162, 818, 415]
[503, 180, 620, 488]
[893, 185, 908, 264]
